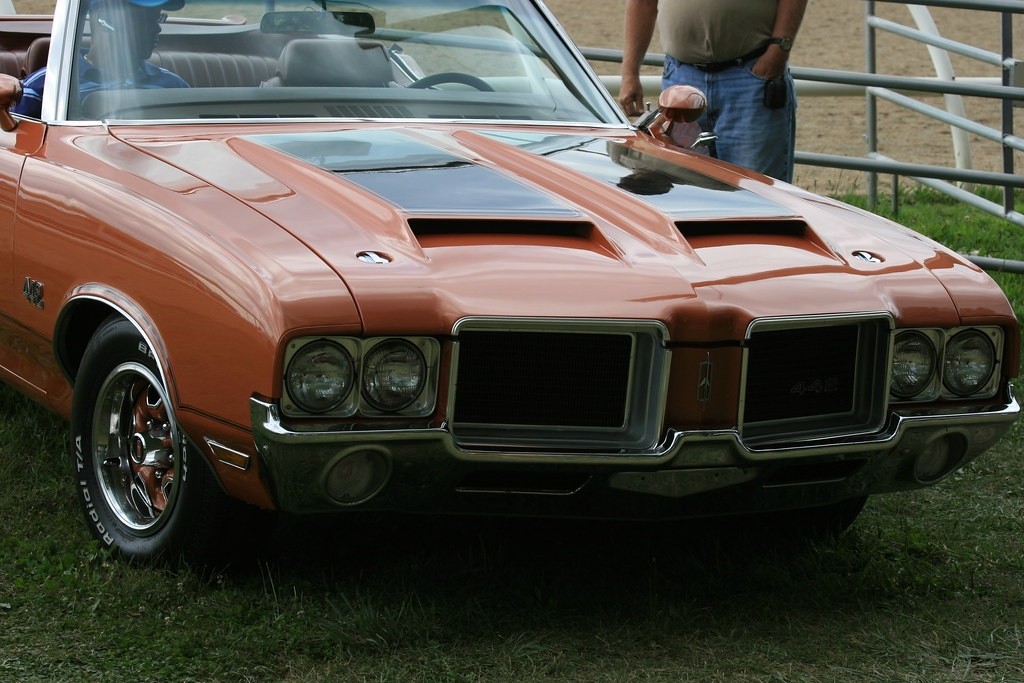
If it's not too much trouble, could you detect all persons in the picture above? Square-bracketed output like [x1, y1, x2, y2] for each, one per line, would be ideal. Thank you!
[11, 0, 192, 119]
[618, 0, 808, 183]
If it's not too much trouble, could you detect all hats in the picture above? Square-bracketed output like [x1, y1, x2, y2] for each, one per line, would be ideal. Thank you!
[87, 0, 186, 11]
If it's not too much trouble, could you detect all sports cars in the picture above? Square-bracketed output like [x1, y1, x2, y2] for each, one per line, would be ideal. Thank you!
[0, 1, 1023, 571]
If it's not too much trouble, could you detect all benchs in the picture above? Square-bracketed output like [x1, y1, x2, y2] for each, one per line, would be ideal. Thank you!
[0, 47, 278, 87]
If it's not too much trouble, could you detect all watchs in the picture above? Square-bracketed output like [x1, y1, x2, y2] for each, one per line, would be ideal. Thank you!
[767, 37, 792, 51]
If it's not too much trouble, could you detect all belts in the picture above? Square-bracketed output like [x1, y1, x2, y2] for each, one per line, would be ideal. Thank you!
[680, 44, 769, 74]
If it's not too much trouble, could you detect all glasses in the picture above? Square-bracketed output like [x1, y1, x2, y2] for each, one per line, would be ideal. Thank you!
[135, 10, 167, 24]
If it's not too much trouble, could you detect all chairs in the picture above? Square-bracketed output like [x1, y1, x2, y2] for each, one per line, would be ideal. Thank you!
[263, 38, 397, 87]
[22, 37, 90, 78]
